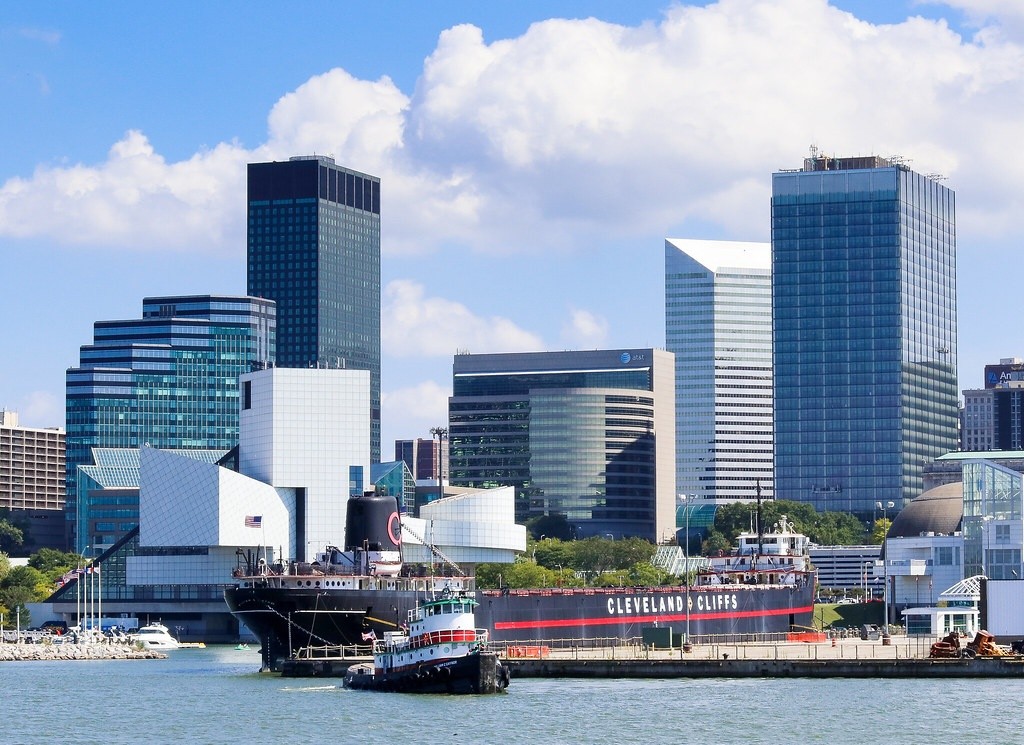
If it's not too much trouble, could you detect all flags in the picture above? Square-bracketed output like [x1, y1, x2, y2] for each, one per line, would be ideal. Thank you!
[68, 567, 100, 578]
[362, 630, 376, 641]
[244, 515, 262, 528]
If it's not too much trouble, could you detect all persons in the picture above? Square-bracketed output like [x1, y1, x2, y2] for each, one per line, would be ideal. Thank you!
[402, 620, 408, 637]
[51, 628, 61, 636]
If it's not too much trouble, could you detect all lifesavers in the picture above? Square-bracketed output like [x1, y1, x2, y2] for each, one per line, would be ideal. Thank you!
[441, 667, 450, 678]
[420, 671, 429, 683]
[504, 671, 509, 685]
[786, 548, 791, 554]
[412, 672, 419, 689]
[351, 674, 412, 692]
[430, 666, 440, 680]
[502, 666, 509, 680]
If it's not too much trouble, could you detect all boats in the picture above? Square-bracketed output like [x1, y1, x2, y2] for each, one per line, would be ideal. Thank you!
[127, 616, 178, 651]
[340, 512, 511, 694]
[369, 560, 403, 578]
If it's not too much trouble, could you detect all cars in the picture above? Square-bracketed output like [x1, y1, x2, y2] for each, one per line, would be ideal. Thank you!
[814, 597, 833, 604]
[837, 598, 859, 604]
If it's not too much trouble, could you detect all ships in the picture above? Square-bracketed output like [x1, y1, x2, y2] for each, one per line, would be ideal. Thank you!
[222, 483, 821, 674]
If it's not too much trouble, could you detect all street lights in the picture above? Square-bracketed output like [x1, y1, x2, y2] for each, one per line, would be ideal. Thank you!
[876, 500, 895, 645]
[678, 492, 697, 652]
[865, 562, 871, 603]
[860, 554, 862, 604]
